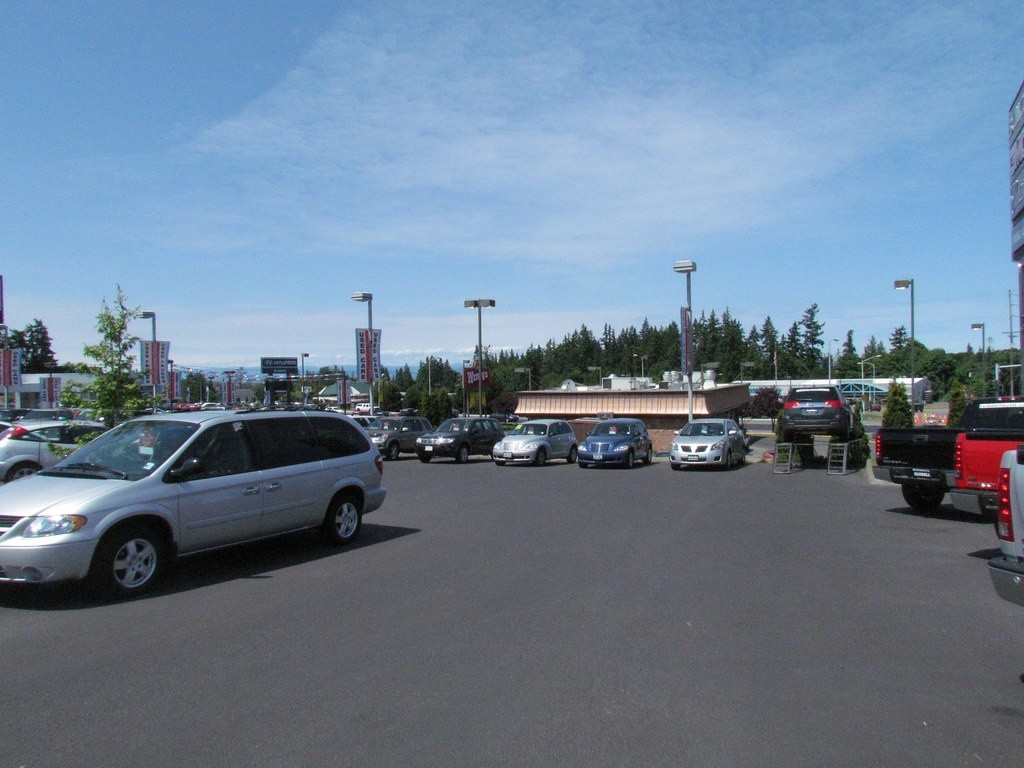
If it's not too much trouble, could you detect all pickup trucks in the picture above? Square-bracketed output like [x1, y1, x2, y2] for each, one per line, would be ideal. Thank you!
[947, 427, 1024, 520]
[986, 440, 1024, 580]
[870, 395, 1024, 512]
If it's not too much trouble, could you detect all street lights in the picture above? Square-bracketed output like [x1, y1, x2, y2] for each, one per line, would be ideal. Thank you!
[166, 360, 174, 409]
[827, 338, 839, 385]
[586, 367, 602, 386]
[350, 291, 375, 416]
[673, 259, 697, 421]
[514, 367, 532, 391]
[971, 323, 986, 364]
[856, 361, 877, 406]
[860, 354, 882, 412]
[134, 311, 159, 414]
[207, 376, 215, 382]
[222, 370, 236, 405]
[464, 299, 496, 418]
[428, 355, 440, 396]
[895, 277, 916, 424]
[463, 359, 471, 418]
[300, 351, 309, 405]
[633, 353, 645, 376]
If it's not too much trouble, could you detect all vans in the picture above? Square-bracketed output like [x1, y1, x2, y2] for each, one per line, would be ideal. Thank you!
[356, 402, 372, 412]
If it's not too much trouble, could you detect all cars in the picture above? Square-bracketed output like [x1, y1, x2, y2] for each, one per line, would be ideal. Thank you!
[324, 406, 344, 414]
[488, 411, 519, 422]
[669, 418, 747, 471]
[0, 407, 170, 482]
[779, 383, 856, 440]
[345, 415, 382, 434]
[491, 418, 579, 468]
[577, 418, 653, 469]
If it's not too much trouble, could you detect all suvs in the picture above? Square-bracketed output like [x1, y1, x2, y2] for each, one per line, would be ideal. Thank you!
[0, 404, 387, 599]
[411, 416, 507, 462]
[365, 408, 434, 461]
